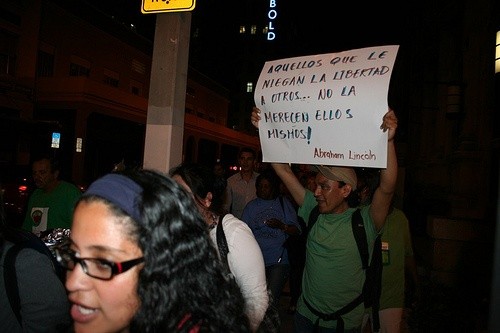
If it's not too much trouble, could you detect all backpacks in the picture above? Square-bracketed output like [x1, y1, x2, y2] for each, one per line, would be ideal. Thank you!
[300, 204, 382, 333]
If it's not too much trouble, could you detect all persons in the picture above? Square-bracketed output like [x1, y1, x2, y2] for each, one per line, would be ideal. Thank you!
[251, 106, 398, 333]
[21, 148, 80, 237]
[0, 204, 72, 333]
[214, 163, 227, 177]
[348, 168, 379, 208]
[240, 174, 304, 333]
[56, 171, 252, 333]
[287, 172, 320, 317]
[357, 182, 423, 333]
[223, 148, 262, 220]
[169, 158, 269, 333]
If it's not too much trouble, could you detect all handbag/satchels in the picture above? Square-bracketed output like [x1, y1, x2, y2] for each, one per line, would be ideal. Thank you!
[256, 293, 286, 333]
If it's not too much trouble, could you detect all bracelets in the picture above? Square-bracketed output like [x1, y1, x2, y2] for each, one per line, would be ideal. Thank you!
[283, 224, 288, 232]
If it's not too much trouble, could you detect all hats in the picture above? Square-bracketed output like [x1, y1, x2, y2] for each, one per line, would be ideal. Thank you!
[314, 165, 358, 192]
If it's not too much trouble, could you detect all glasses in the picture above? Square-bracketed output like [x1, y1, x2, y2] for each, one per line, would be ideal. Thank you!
[53, 245, 144, 280]
